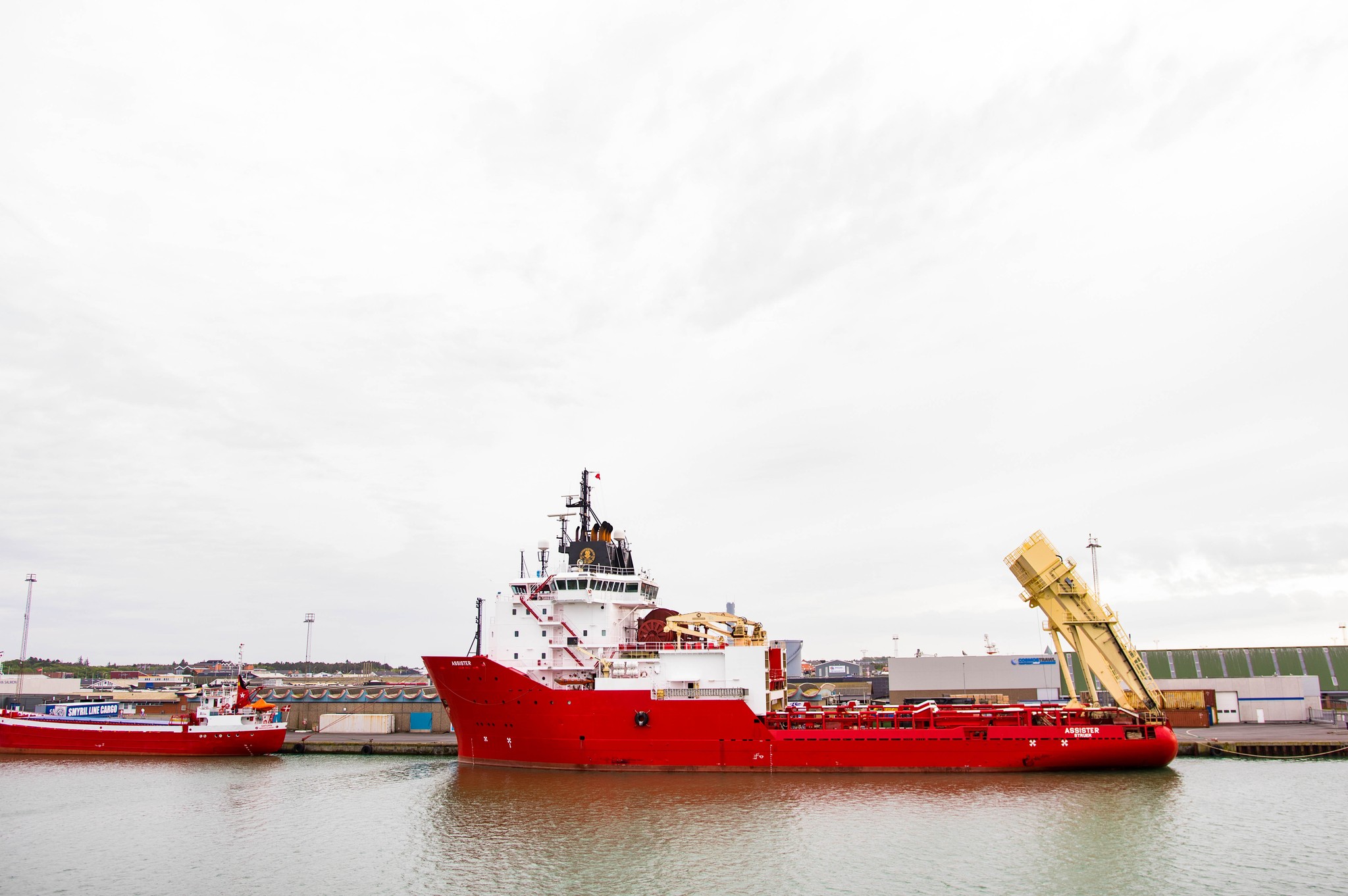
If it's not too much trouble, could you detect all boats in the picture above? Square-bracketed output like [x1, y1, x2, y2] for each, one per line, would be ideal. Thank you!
[0, 643, 293, 755]
[424, 470, 1180, 774]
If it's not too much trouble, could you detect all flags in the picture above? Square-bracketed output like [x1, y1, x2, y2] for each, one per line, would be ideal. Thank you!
[595, 472, 600, 480]
[37, 668, 42, 672]
[237, 675, 251, 709]
[280, 705, 291, 712]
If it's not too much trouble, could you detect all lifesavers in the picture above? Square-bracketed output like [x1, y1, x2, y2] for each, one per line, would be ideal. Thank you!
[303, 720, 307, 725]
[538, 596, 542, 600]
[497, 595, 498, 598]
[293, 743, 304, 754]
[635, 711, 648, 726]
[258, 715, 263, 720]
[256, 716, 260, 721]
[1210, 745, 1224, 756]
[219, 709, 224, 714]
[361, 745, 372, 754]
[264, 720, 267, 724]
[225, 703, 230, 709]
[641, 671, 647, 676]
